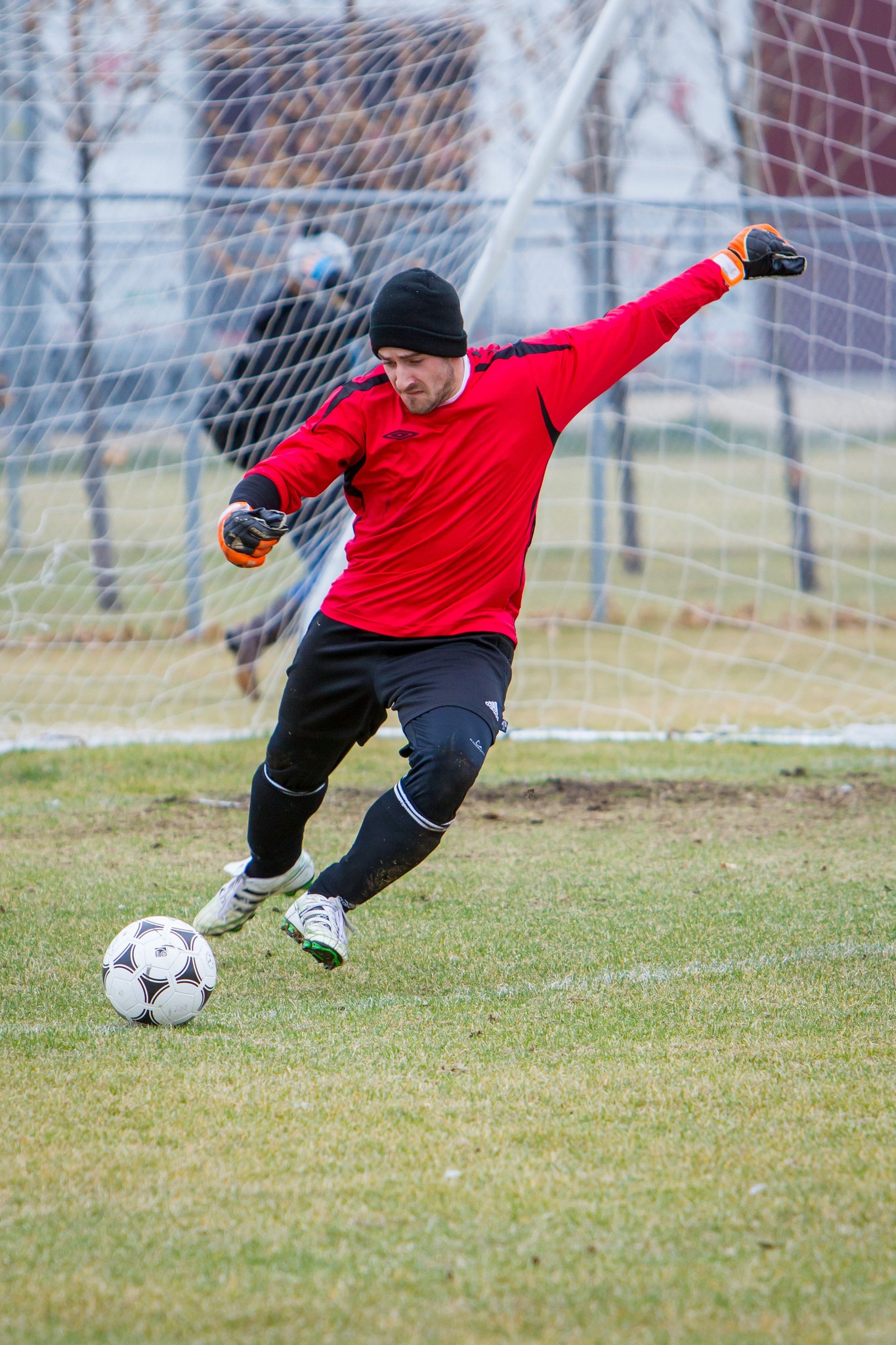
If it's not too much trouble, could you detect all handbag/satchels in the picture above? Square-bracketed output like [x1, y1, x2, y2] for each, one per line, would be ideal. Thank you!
[198, 354, 301, 471]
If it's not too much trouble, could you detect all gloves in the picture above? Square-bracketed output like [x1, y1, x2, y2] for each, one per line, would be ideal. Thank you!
[710, 223, 807, 288]
[216, 501, 290, 569]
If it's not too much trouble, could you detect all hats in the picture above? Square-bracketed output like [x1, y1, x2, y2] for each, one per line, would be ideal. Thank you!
[368, 266, 468, 359]
[286, 223, 353, 302]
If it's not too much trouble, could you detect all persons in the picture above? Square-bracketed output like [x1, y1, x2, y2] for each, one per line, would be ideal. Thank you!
[196, 224, 374, 698]
[191, 223, 807, 970]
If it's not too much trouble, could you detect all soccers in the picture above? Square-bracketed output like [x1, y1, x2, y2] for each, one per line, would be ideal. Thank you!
[100, 914, 221, 1028]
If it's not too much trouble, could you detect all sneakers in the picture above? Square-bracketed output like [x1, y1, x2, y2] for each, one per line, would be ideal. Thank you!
[223, 630, 265, 702]
[192, 849, 316, 939]
[280, 890, 359, 974]
